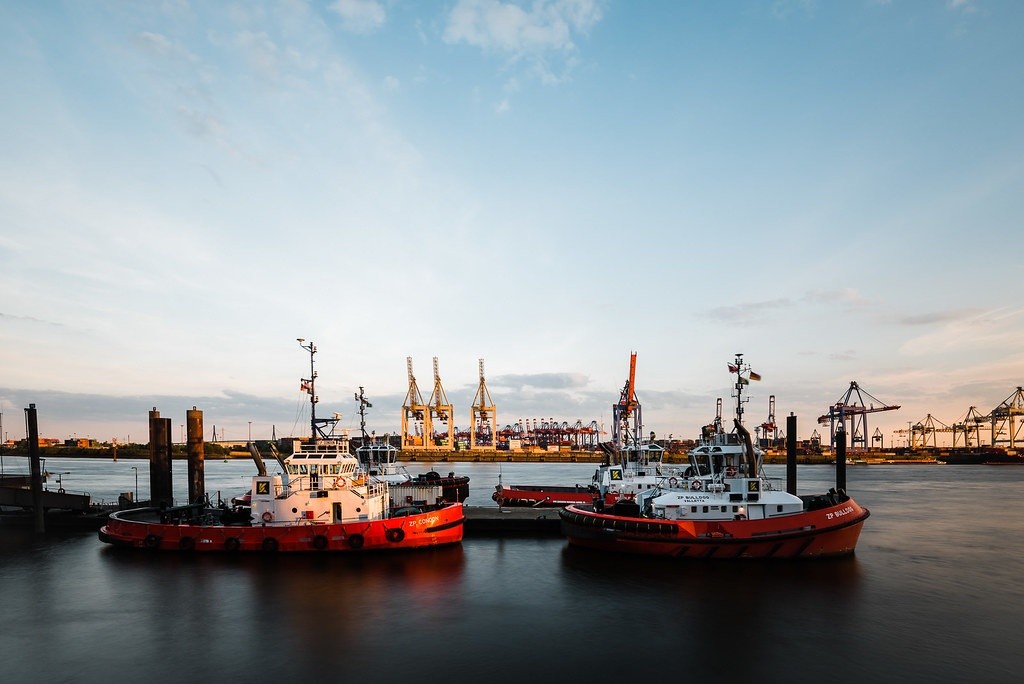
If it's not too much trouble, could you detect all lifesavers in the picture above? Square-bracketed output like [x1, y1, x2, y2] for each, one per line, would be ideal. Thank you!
[733, 513, 747, 521]
[390, 528, 404, 542]
[144, 534, 160, 548]
[313, 535, 327, 548]
[349, 534, 364, 548]
[262, 537, 278, 554]
[727, 467, 736, 476]
[58, 488, 65, 493]
[224, 537, 240, 552]
[669, 477, 677, 485]
[336, 478, 346, 487]
[692, 481, 701, 490]
[180, 536, 195, 551]
[358, 474, 367, 482]
[262, 512, 272, 522]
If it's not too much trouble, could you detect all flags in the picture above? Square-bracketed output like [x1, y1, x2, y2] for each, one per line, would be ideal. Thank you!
[739, 377, 748, 385]
[750, 372, 760, 380]
[729, 366, 738, 373]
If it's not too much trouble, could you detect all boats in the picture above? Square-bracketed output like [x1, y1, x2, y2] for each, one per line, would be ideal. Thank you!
[936, 460, 947, 465]
[232, 386, 472, 507]
[832, 458, 855, 465]
[96, 338, 469, 555]
[493, 351, 687, 508]
[559, 354, 871, 561]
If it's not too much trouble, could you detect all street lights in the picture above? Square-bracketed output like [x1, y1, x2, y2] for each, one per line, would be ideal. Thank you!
[49, 472, 70, 489]
[130, 466, 138, 502]
